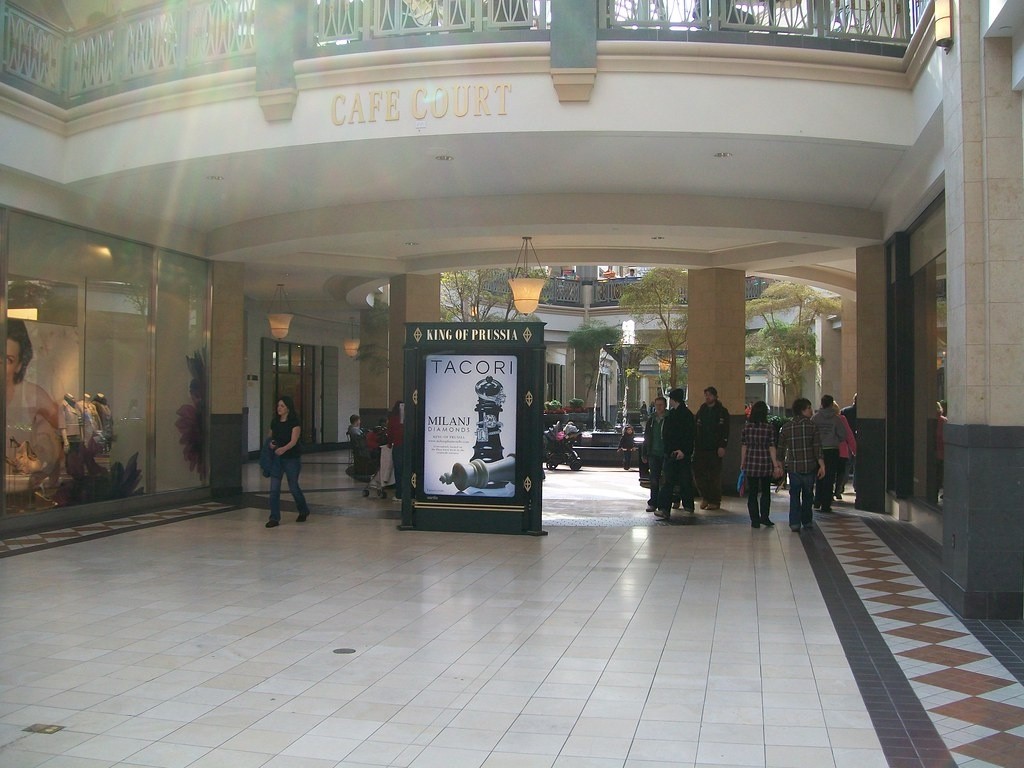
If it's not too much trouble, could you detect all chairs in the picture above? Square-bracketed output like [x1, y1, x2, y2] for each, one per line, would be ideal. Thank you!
[347, 433, 366, 464]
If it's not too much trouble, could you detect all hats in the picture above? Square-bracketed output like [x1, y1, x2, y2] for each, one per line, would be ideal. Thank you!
[665, 388, 685, 402]
[704, 387, 717, 396]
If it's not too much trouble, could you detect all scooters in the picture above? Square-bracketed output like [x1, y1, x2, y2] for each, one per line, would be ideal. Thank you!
[544, 420, 583, 471]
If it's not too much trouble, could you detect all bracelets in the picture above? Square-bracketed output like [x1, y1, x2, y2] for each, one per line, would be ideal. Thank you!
[741, 464, 745, 466]
[774, 466, 779, 468]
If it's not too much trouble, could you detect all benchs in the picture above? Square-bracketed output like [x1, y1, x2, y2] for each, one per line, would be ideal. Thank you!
[570, 441, 644, 466]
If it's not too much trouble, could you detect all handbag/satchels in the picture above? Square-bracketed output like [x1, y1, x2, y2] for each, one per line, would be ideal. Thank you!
[737, 470, 748, 497]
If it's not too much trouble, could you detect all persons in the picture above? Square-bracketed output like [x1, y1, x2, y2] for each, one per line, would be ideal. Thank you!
[618, 425, 636, 470]
[6, 316, 58, 478]
[740, 392, 857, 532]
[694, 387, 730, 511]
[626, 269, 636, 278]
[348, 401, 404, 501]
[564, 270, 579, 280]
[566, 422, 577, 447]
[60, 394, 113, 455]
[265, 396, 310, 528]
[642, 387, 696, 519]
[935, 400, 947, 503]
[610, 271, 616, 279]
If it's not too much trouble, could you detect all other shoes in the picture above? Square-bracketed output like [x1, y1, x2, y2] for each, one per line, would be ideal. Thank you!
[654, 511, 670, 519]
[803, 521, 815, 528]
[646, 505, 656, 512]
[673, 503, 679, 509]
[820, 507, 833, 512]
[689, 508, 694, 512]
[296, 512, 310, 522]
[761, 519, 775, 525]
[836, 496, 842, 499]
[750, 521, 760, 528]
[790, 524, 801, 532]
[685, 506, 690, 511]
[813, 506, 821, 511]
[700, 500, 721, 510]
[392, 496, 402, 502]
[265, 520, 279, 528]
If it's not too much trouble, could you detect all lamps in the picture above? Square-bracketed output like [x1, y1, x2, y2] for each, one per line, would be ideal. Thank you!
[508, 236, 548, 317]
[343, 316, 362, 357]
[267, 284, 294, 341]
